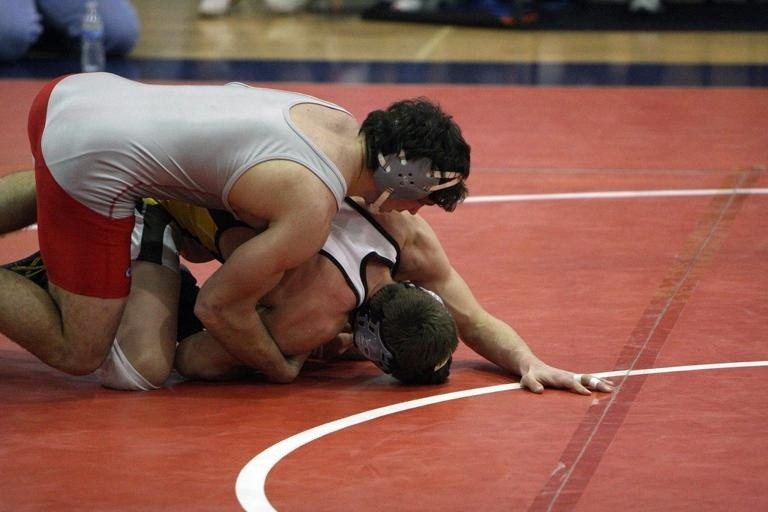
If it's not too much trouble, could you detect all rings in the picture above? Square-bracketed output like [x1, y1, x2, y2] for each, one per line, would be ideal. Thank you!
[587, 376, 602, 392]
[575, 372, 585, 385]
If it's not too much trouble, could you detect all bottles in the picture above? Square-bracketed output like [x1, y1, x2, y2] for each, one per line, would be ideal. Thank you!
[81, 2, 104, 72]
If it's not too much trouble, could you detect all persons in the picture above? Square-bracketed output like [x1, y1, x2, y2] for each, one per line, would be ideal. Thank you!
[1, 68, 472, 377]
[197, 0, 304, 16]
[1, 193, 614, 398]
[0, 0, 143, 64]
